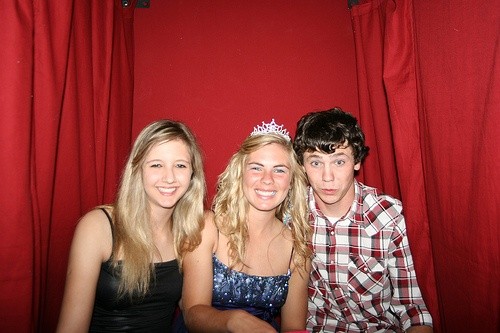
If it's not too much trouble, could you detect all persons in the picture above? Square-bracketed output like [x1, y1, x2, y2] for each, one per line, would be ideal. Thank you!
[182, 119, 316, 333]
[57, 119, 208, 333]
[292, 106, 433, 333]
